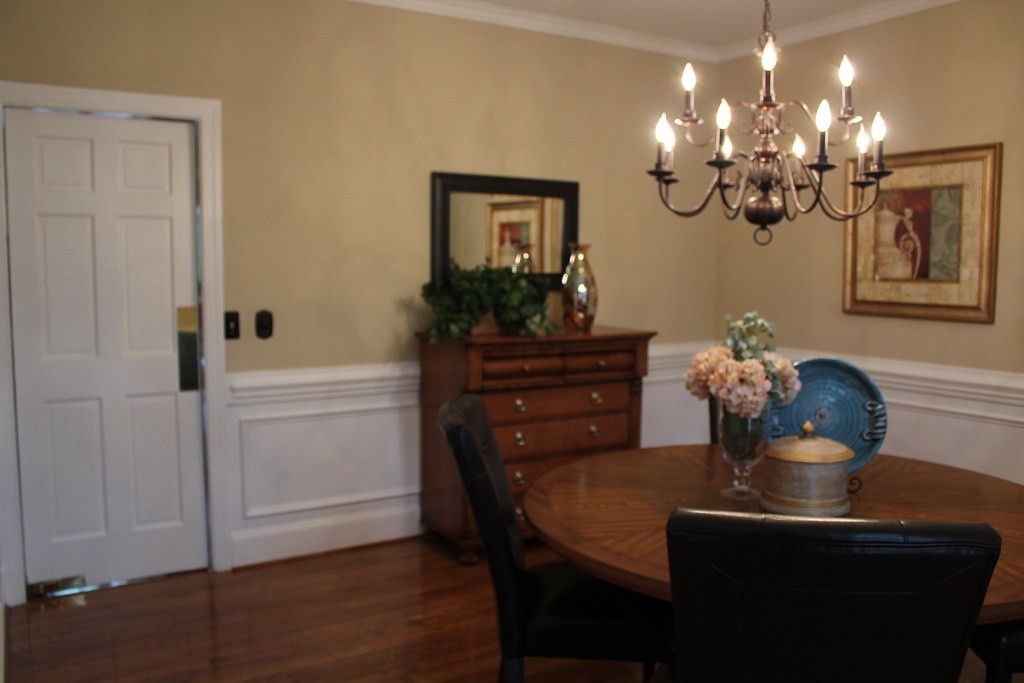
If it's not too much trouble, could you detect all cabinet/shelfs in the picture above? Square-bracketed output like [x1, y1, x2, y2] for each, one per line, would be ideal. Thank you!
[415, 322, 659, 567]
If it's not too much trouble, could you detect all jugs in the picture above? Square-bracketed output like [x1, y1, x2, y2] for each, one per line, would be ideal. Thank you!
[873, 199, 922, 283]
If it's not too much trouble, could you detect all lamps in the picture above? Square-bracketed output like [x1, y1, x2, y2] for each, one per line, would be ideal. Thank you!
[639, 0, 894, 244]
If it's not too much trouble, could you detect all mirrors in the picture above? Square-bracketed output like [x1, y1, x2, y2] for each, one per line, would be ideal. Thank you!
[427, 171, 581, 290]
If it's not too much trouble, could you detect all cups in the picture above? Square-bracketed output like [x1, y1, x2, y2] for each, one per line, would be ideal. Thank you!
[756, 421, 856, 520]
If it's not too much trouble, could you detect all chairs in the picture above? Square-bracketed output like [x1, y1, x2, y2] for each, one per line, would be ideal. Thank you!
[433, 393, 674, 683]
[664, 507, 1001, 683]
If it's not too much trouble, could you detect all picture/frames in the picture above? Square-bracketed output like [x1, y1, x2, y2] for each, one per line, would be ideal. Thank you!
[842, 141, 1003, 325]
[484, 200, 544, 273]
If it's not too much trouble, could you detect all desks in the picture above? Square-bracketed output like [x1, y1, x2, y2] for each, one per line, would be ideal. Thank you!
[519, 444, 1024, 625]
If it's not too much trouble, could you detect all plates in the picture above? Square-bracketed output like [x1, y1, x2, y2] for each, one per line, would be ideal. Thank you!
[764, 357, 888, 480]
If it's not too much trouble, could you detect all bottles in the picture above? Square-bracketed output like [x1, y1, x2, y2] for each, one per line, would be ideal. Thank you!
[509, 243, 534, 277]
[561, 242, 600, 333]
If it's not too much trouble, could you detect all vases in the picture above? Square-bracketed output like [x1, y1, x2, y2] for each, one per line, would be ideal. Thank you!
[720, 399, 772, 501]
[561, 243, 598, 333]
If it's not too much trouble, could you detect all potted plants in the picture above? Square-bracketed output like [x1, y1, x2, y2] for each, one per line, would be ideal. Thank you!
[418, 252, 513, 340]
[487, 266, 557, 339]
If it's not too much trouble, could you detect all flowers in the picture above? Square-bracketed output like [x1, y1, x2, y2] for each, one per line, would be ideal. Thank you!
[682, 308, 801, 419]
